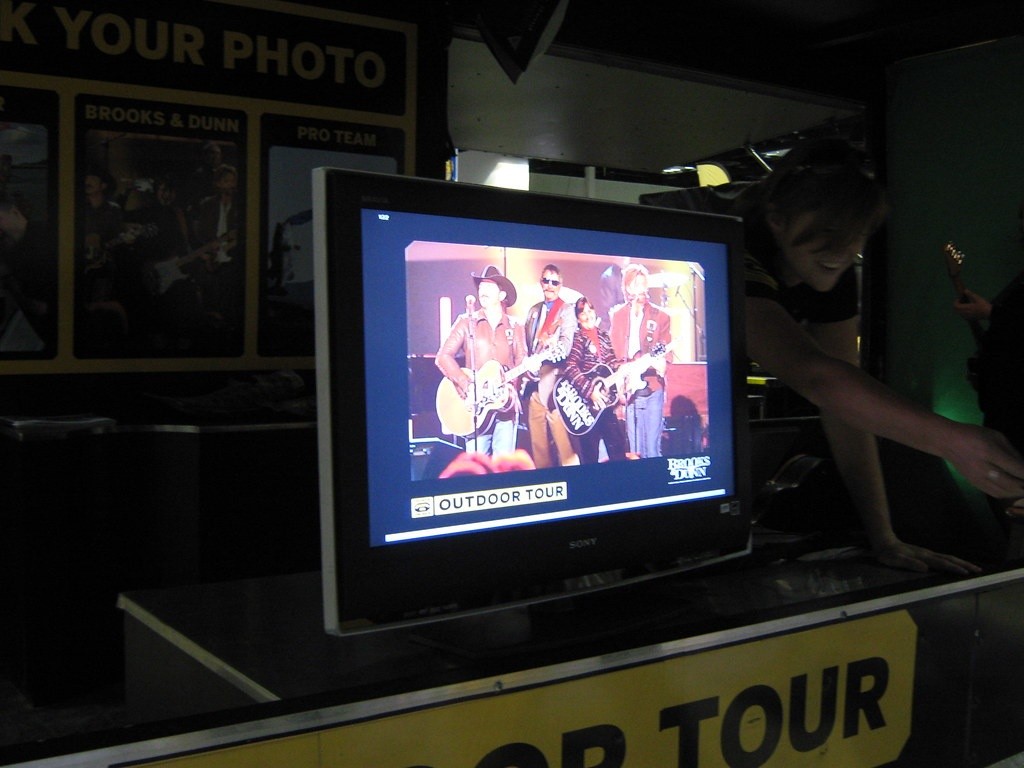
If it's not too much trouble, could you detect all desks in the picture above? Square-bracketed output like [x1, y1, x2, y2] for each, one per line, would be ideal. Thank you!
[100, 531, 968, 731]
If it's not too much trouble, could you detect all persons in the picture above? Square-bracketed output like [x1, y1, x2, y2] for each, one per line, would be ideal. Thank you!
[435, 263, 672, 464]
[735, 135, 1024, 577]
[1, 145, 244, 330]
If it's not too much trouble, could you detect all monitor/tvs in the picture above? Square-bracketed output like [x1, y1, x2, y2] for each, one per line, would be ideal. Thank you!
[310, 166, 753, 659]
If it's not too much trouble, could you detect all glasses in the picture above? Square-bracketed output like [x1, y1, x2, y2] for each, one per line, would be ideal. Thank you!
[541, 277, 561, 287]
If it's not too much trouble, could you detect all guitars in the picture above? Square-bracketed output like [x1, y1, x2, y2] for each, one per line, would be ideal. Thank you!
[436, 341, 567, 440]
[551, 342, 665, 436]
[149, 227, 238, 295]
[86, 224, 159, 271]
[941, 238, 986, 387]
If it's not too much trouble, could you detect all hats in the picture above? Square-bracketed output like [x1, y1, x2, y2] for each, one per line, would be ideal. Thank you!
[470, 266, 517, 308]
